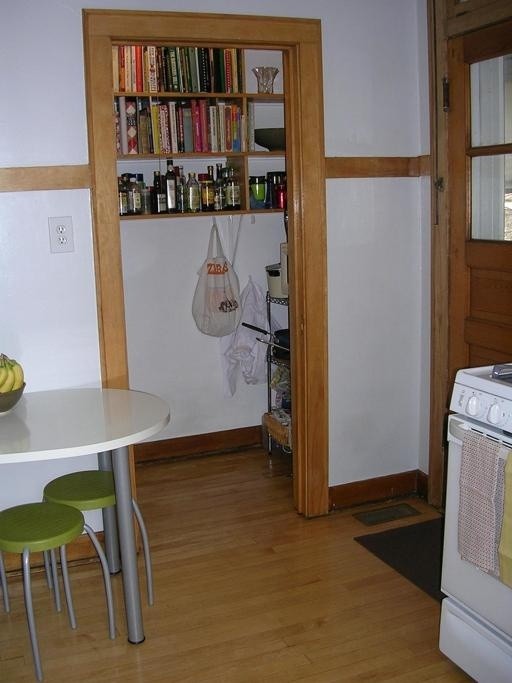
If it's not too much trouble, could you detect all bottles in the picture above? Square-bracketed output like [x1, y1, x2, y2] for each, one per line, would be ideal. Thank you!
[248, 175, 268, 201]
[276, 183, 287, 208]
[117, 157, 242, 215]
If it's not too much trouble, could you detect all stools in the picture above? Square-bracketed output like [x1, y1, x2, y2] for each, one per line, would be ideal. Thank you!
[0, 503, 115, 681]
[42, 471, 153, 627]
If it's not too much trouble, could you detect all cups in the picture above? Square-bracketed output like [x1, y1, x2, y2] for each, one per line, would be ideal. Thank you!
[252, 65, 279, 94]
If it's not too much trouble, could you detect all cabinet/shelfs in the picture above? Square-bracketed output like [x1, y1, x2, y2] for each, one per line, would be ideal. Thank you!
[112, 45, 285, 220]
[265, 290, 291, 457]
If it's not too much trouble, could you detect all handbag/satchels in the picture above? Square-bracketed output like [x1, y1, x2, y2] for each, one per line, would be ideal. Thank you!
[189, 223, 243, 337]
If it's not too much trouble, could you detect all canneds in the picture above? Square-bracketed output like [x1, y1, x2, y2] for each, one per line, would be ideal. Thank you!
[201, 180, 216, 211]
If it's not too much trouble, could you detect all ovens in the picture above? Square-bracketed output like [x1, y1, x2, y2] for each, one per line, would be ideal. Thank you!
[438, 412, 511, 683]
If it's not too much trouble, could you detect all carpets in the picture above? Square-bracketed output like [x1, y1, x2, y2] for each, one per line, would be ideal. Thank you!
[354, 518, 443, 603]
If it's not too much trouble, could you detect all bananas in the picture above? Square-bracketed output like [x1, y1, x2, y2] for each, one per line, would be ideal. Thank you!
[0, 353, 23, 395]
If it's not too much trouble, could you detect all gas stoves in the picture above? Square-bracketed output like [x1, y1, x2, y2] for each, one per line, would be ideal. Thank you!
[448, 362, 511, 435]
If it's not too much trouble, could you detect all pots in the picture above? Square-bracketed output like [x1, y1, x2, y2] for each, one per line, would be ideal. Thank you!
[241, 322, 290, 360]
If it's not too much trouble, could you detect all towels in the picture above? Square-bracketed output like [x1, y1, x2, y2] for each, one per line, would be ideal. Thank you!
[457, 433, 512, 590]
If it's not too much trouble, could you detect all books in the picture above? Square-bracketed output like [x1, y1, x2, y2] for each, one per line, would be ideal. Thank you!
[113, 42, 256, 156]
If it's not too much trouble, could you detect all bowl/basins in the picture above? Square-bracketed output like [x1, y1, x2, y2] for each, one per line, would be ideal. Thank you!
[254, 127, 286, 151]
[1, 382, 26, 412]
